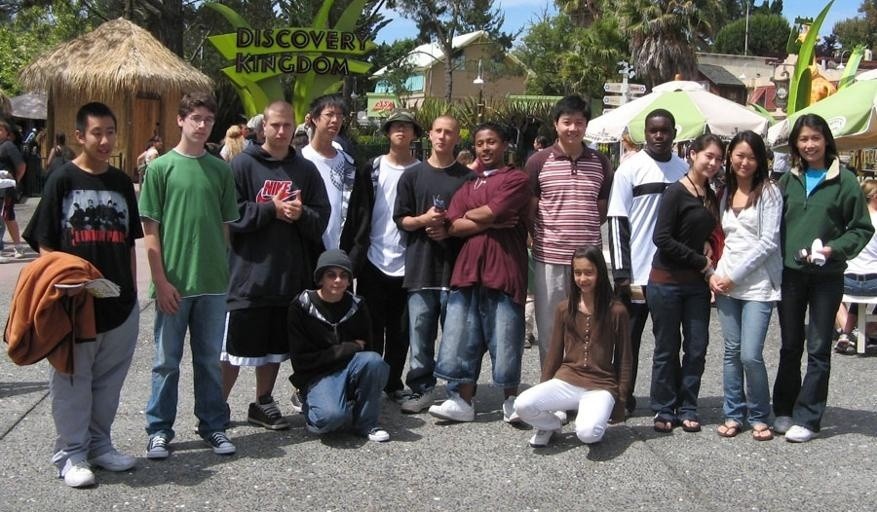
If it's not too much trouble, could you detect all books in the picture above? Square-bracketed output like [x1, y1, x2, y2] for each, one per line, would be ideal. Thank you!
[52, 277, 122, 300]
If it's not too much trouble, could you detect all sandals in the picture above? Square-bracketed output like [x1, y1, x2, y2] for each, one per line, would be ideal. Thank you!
[652, 413, 700, 432]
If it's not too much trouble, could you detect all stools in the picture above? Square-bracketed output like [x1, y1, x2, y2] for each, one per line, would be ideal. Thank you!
[841, 293, 877, 356]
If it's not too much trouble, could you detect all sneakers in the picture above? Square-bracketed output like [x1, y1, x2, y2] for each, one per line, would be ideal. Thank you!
[428, 392, 475, 423]
[1, 245, 26, 263]
[833, 326, 870, 354]
[776, 413, 820, 442]
[504, 397, 568, 448]
[86, 448, 138, 472]
[193, 400, 236, 454]
[247, 394, 290, 430]
[145, 434, 170, 459]
[362, 421, 390, 442]
[523, 333, 536, 349]
[390, 384, 439, 411]
[289, 389, 305, 414]
[64, 459, 96, 488]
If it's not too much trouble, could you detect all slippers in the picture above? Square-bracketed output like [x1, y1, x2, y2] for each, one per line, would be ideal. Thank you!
[716, 422, 741, 438]
[751, 425, 774, 441]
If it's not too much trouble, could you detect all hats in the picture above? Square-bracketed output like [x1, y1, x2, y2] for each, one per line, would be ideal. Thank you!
[379, 109, 424, 136]
[313, 248, 354, 288]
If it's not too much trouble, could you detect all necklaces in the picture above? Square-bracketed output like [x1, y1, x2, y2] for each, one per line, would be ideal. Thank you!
[685, 175, 706, 206]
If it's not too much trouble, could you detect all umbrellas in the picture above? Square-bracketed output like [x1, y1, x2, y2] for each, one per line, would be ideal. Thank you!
[763, 68, 875, 152]
[578, 72, 770, 148]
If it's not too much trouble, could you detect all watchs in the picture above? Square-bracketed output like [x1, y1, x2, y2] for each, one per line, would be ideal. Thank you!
[615, 132, 638, 166]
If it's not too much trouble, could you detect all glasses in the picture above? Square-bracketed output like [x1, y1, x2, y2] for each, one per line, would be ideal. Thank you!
[325, 270, 350, 280]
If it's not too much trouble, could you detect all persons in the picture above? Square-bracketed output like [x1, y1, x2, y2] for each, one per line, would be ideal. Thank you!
[69, 199, 125, 225]
[832, 178, 876, 357]
[457, 147, 473, 169]
[299, 98, 356, 274]
[532, 134, 547, 153]
[138, 90, 237, 460]
[283, 246, 392, 443]
[393, 114, 478, 412]
[22, 127, 38, 151]
[135, 110, 324, 184]
[511, 242, 636, 452]
[19, 100, 146, 487]
[344, 109, 426, 406]
[524, 95, 613, 423]
[642, 132, 723, 437]
[215, 99, 333, 432]
[0, 121, 27, 261]
[426, 124, 533, 426]
[45, 130, 76, 169]
[767, 147, 795, 185]
[770, 112, 875, 442]
[834, 301, 874, 355]
[525, 246, 536, 350]
[704, 128, 783, 442]
[6, 126, 21, 148]
[602, 107, 690, 423]
